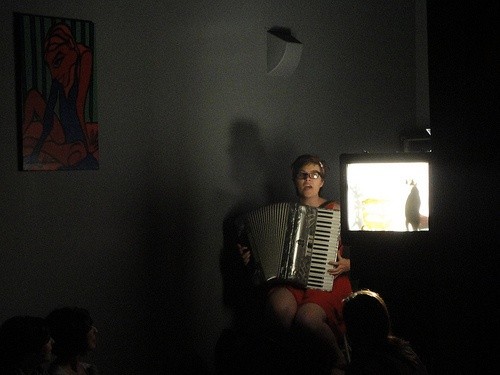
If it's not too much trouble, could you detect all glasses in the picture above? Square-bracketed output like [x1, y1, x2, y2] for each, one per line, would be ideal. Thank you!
[294, 169, 324, 181]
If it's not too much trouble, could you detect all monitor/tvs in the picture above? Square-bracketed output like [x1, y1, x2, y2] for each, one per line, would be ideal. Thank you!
[339, 152, 437, 245]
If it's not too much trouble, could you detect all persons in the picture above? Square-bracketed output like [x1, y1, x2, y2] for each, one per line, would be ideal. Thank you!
[342, 289, 426, 375]
[237, 155, 353, 375]
[0, 306, 100, 375]
